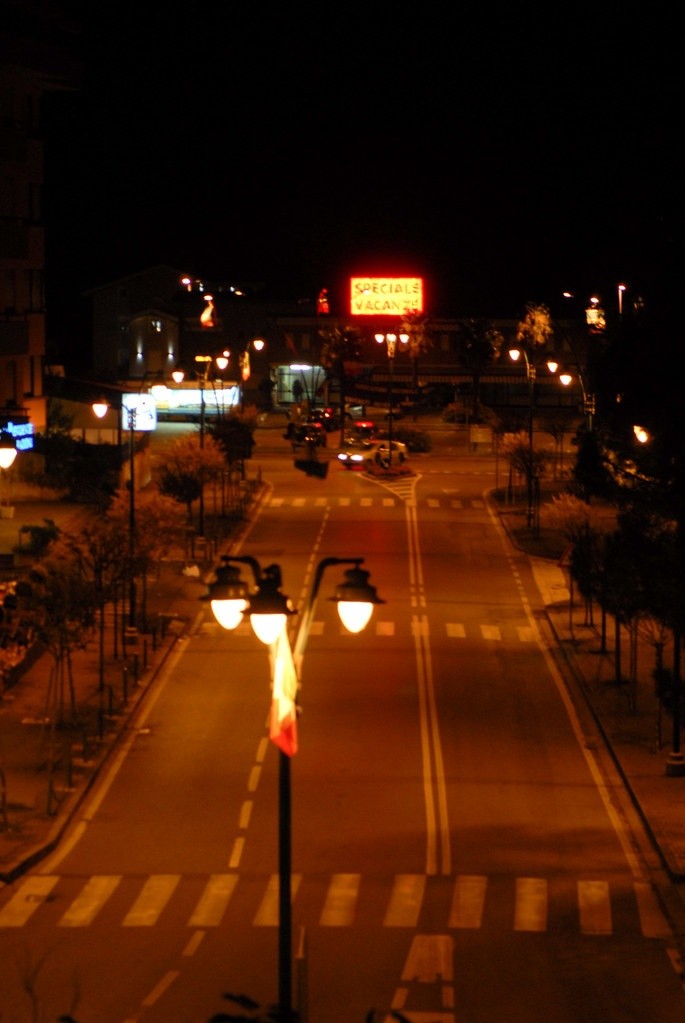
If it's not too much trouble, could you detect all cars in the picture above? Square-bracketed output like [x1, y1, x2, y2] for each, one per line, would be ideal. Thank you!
[284, 407, 372, 448]
[338, 438, 408, 472]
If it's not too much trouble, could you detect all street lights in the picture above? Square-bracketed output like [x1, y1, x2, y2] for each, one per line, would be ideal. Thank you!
[193, 552, 385, 1021]
[373, 333, 409, 467]
[173, 362, 205, 536]
[632, 422, 682, 754]
[216, 340, 264, 483]
[92, 400, 141, 643]
[506, 348, 573, 531]
